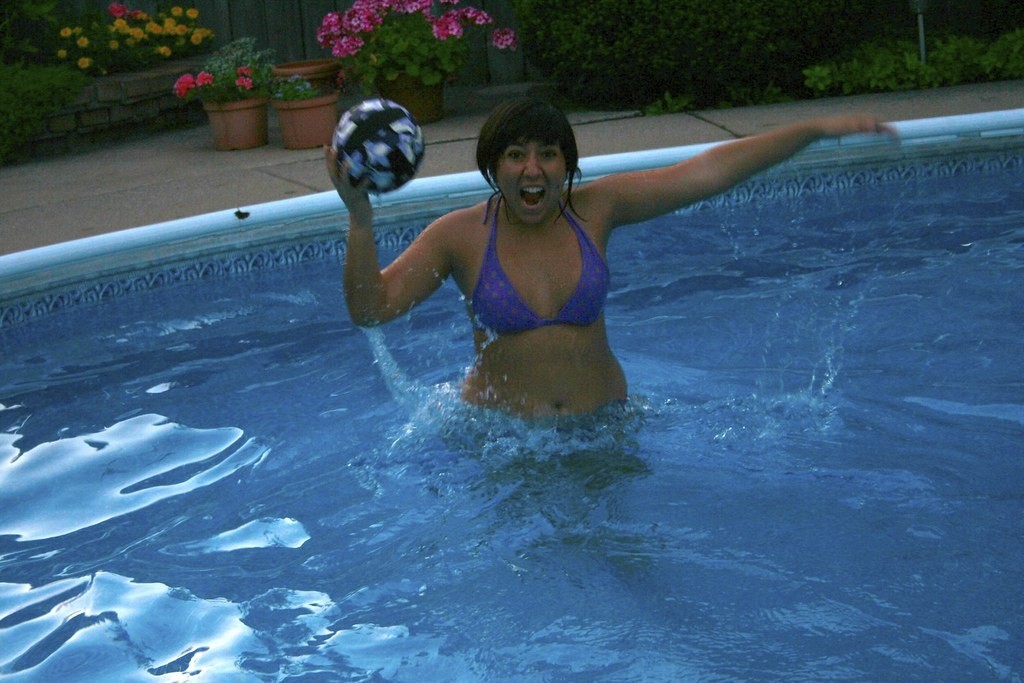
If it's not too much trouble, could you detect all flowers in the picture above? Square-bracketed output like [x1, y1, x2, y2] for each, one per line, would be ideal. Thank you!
[171, 37, 273, 104]
[314, 0, 521, 93]
[266, 75, 333, 101]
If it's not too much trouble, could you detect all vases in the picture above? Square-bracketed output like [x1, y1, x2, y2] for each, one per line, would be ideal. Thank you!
[203, 97, 270, 151]
[274, 59, 337, 92]
[379, 70, 446, 124]
[271, 90, 339, 148]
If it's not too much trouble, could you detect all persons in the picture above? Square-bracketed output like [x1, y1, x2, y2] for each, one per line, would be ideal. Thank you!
[325, 98, 901, 428]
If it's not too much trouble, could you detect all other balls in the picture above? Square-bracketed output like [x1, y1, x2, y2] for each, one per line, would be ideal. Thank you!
[329, 97, 425, 195]
